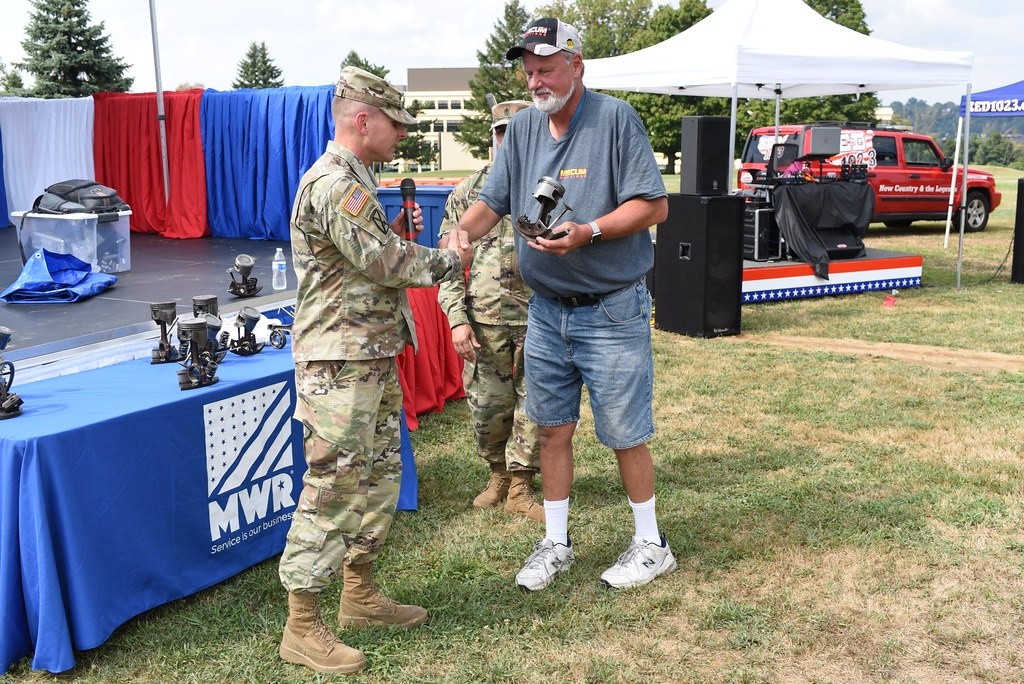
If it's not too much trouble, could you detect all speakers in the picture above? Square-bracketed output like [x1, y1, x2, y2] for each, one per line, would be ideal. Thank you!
[646, 115, 745, 340]
[811, 227, 864, 260]
[1011, 178, 1024, 283]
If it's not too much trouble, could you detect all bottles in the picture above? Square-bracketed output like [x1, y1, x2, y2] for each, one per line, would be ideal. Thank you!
[271, 248, 287, 289]
[802, 163, 811, 175]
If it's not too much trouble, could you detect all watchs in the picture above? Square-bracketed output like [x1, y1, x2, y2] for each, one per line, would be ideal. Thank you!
[588, 221, 603, 244]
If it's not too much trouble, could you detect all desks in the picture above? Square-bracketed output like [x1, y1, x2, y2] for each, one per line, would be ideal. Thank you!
[746, 182, 874, 261]
[0, 331, 421, 669]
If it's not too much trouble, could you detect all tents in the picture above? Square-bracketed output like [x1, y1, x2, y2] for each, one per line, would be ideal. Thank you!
[581, 2, 1024, 290]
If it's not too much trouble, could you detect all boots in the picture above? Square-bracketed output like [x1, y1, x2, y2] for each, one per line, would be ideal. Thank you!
[503, 471, 548, 523]
[339, 562, 428, 629]
[473, 461, 512, 509]
[279, 592, 367, 674]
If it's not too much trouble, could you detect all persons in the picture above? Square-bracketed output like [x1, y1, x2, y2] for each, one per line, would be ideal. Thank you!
[276, 65, 475, 675]
[437, 99, 546, 523]
[437, 16, 677, 593]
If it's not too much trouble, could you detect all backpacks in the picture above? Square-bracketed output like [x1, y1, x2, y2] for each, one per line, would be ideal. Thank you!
[32, 179, 131, 213]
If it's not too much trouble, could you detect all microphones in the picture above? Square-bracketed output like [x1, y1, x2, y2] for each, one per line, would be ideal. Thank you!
[401, 178, 418, 244]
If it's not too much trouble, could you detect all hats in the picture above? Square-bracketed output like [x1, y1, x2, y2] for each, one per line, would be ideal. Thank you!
[506, 17, 582, 61]
[489, 100, 534, 131]
[334, 66, 419, 125]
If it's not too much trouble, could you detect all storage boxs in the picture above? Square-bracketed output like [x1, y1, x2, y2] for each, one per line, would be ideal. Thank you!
[376, 184, 456, 249]
[9, 209, 132, 276]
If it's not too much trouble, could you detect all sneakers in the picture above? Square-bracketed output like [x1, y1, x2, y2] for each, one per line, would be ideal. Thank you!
[515, 531, 576, 592]
[600, 532, 678, 592]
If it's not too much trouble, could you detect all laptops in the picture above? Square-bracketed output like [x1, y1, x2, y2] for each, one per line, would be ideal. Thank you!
[796, 128, 841, 162]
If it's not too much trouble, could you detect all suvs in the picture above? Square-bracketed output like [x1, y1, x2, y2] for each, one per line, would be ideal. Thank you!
[737, 120, 1002, 232]
